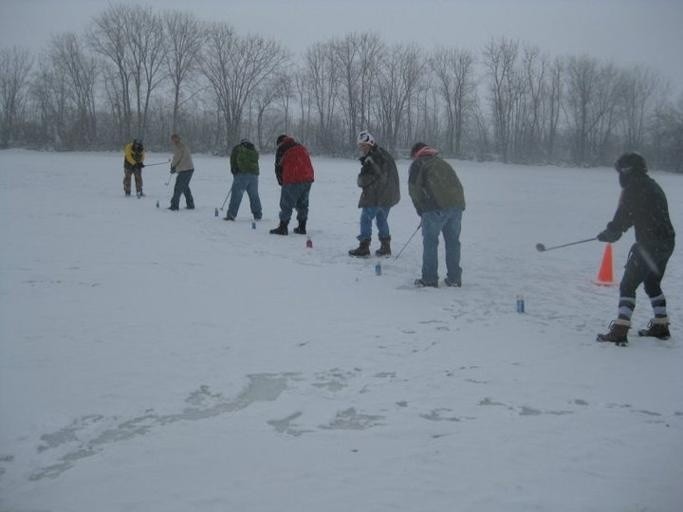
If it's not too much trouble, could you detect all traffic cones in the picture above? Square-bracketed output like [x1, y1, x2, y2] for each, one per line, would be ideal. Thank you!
[590, 242, 618, 287]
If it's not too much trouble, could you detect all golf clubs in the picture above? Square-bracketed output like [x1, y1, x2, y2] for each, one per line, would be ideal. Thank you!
[144, 159, 170, 166]
[163, 173, 172, 187]
[218, 187, 232, 210]
[535, 237, 600, 251]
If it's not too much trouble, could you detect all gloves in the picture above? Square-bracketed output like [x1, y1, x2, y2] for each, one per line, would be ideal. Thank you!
[598, 228, 622, 242]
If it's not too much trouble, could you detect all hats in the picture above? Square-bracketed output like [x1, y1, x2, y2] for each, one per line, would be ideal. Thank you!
[615, 152, 647, 186]
[357, 131, 375, 146]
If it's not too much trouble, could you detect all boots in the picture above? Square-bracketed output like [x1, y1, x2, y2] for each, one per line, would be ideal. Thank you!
[349, 239, 370, 256]
[269, 221, 289, 235]
[376, 238, 390, 255]
[639, 318, 669, 337]
[597, 320, 630, 342]
[293, 221, 306, 233]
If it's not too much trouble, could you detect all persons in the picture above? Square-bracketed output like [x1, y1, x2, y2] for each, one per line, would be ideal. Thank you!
[162, 134, 195, 211]
[346, 129, 401, 259]
[268, 135, 314, 236]
[594, 151, 673, 345]
[407, 142, 466, 288]
[122, 138, 147, 198]
[221, 139, 262, 222]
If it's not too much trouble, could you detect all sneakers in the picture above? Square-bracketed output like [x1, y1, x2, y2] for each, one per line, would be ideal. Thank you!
[445, 279, 460, 287]
[416, 280, 437, 286]
